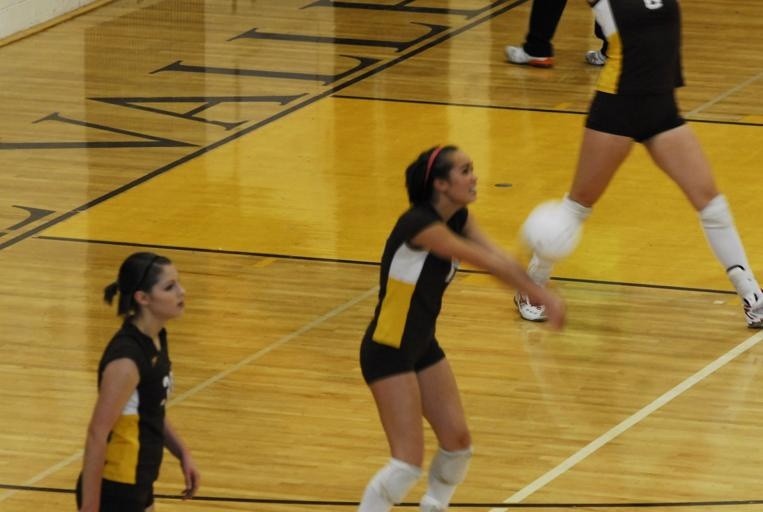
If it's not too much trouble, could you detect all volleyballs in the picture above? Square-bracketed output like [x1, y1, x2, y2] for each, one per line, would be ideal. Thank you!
[522, 200, 581, 259]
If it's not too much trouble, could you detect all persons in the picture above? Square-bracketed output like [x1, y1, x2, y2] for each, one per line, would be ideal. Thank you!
[502, 0, 609, 68]
[511, 2, 762, 330]
[354, 143, 568, 511]
[75, 250, 201, 511]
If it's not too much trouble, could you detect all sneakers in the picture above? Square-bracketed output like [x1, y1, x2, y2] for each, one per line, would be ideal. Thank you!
[742, 291, 763, 332]
[512, 290, 549, 323]
[583, 47, 609, 68]
[503, 42, 557, 69]
